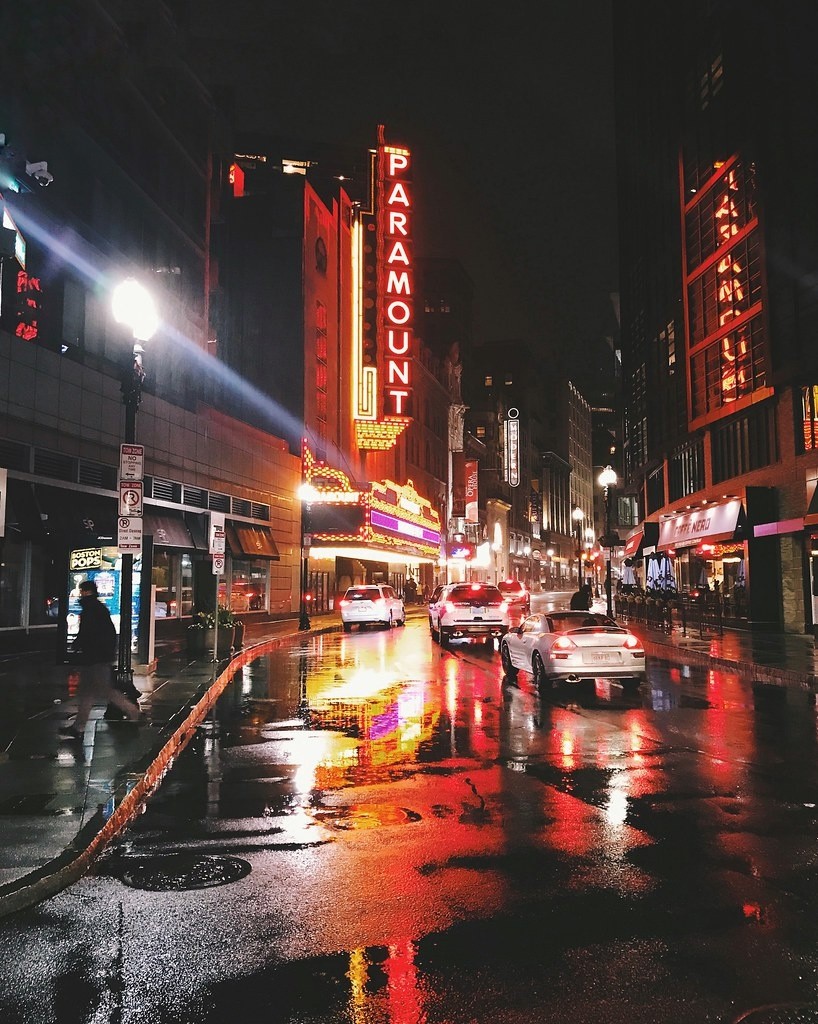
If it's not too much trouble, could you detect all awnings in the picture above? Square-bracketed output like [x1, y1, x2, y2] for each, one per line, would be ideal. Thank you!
[225, 524, 281, 562]
[143, 515, 196, 554]
[657, 501, 742, 552]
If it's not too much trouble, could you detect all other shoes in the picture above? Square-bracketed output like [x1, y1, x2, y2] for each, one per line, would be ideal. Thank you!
[58, 726, 84, 742]
[137, 712, 148, 743]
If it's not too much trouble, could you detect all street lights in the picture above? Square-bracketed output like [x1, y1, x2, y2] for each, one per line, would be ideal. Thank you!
[598, 463, 620, 623]
[573, 506, 586, 589]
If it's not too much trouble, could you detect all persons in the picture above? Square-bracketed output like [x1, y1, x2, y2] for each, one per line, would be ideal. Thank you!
[570, 585, 592, 611]
[57, 580, 145, 740]
[404, 578, 430, 607]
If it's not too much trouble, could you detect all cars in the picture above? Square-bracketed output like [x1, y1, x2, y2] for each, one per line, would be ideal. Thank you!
[340, 583, 407, 631]
[425, 580, 511, 647]
[500, 611, 647, 692]
[497, 580, 531, 611]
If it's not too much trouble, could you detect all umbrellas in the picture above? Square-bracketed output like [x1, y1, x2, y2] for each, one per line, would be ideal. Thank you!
[621, 551, 675, 591]
